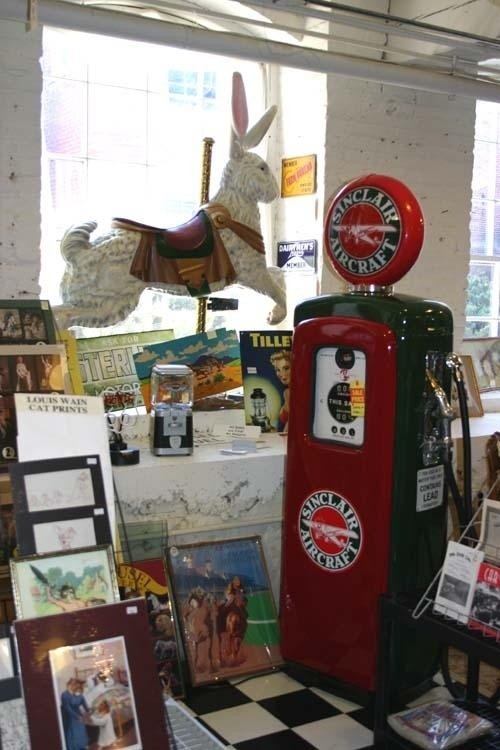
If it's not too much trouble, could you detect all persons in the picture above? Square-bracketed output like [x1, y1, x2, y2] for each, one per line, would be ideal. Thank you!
[88, 699, 119, 749]
[216, 574, 249, 635]
[0, 305, 61, 464]
[270, 351, 291, 433]
[58, 674, 93, 749]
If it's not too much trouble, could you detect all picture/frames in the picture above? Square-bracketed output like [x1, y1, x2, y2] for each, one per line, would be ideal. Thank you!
[12, 596, 169, 750]
[0, 343, 76, 474]
[9, 454, 121, 621]
[0, 298, 57, 345]
[165, 533, 285, 686]
[450, 354, 484, 418]
[461, 336, 500, 392]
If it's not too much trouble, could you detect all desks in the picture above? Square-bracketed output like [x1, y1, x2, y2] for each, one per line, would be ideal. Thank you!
[368, 579, 500, 750]
